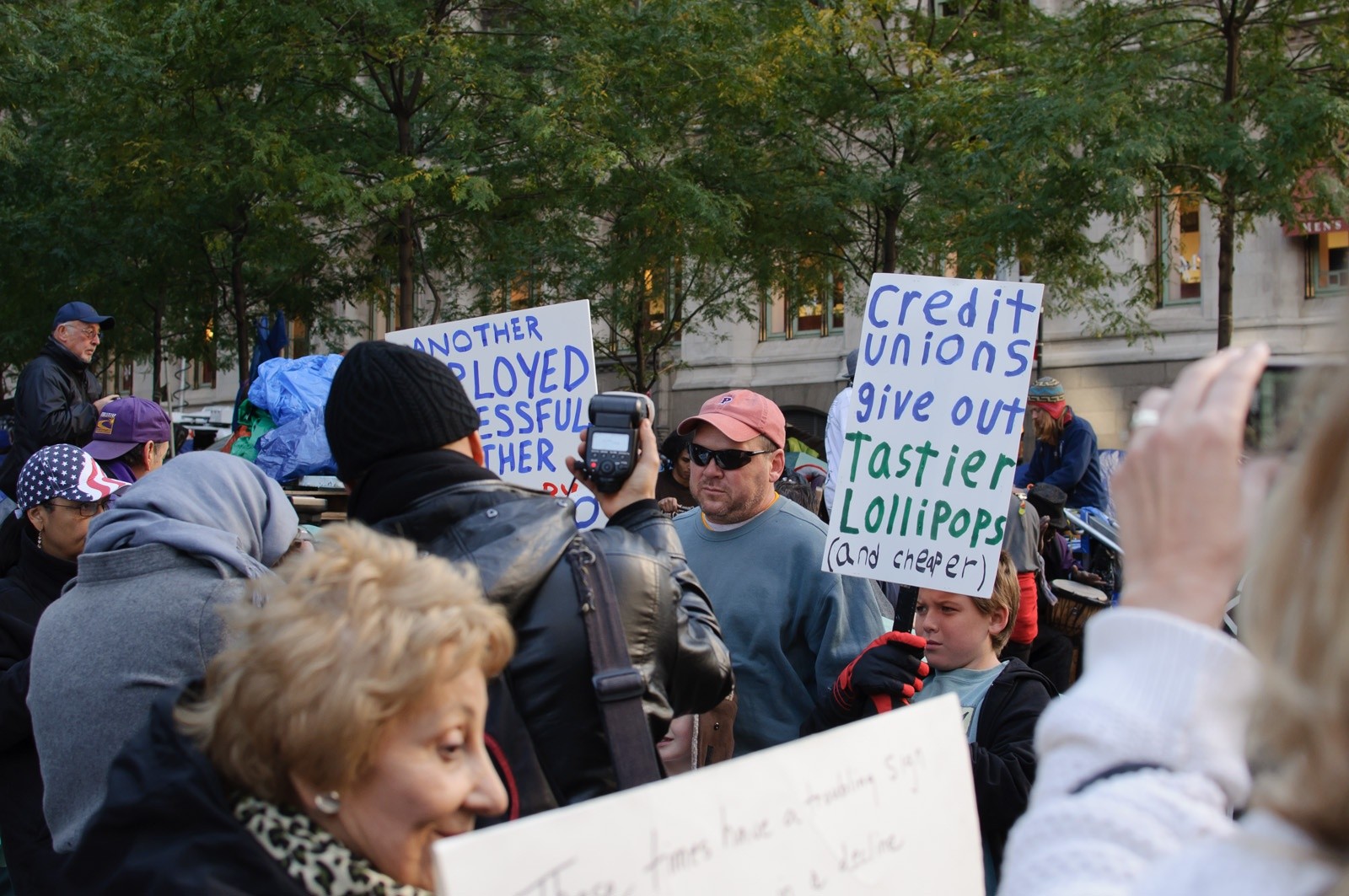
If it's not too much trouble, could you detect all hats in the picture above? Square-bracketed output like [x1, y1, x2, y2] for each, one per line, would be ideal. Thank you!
[676, 388, 786, 448]
[52, 301, 115, 331]
[82, 395, 172, 461]
[1027, 482, 1069, 529]
[14, 443, 133, 520]
[1027, 376, 1072, 425]
[323, 339, 481, 483]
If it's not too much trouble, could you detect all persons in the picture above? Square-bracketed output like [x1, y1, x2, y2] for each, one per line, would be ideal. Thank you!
[26, 447, 328, 888]
[656, 688, 738, 778]
[831, 546, 1062, 896]
[0, 343, 1128, 707]
[15, 299, 124, 486]
[664, 386, 899, 777]
[0, 441, 136, 894]
[991, 328, 1344, 895]
[324, 339, 734, 829]
[58, 520, 519, 896]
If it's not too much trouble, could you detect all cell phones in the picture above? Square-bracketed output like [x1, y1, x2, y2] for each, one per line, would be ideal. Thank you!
[1244, 366, 1346, 448]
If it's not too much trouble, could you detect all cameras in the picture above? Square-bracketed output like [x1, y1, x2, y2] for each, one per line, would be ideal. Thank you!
[112, 396, 121, 400]
[583, 390, 656, 493]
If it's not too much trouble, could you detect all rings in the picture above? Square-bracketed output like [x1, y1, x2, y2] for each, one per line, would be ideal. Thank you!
[1128, 411, 1156, 428]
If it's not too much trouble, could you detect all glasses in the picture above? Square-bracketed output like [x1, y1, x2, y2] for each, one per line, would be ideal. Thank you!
[688, 443, 774, 471]
[42, 500, 115, 517]
[66, 325, 103, 340]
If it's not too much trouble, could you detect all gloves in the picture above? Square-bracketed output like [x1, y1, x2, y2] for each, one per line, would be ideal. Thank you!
[832, 630, 931, 714]
